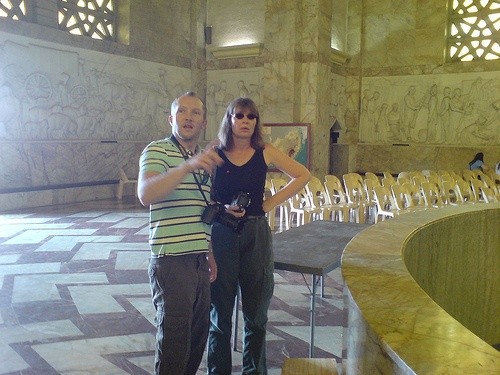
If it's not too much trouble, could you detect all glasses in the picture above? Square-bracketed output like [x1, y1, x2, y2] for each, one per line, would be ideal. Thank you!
[230, 112, 258, 119]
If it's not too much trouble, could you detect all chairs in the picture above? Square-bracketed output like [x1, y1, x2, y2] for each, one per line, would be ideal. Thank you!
[117, 166, 138, 200]
[262, 168, 500, 232]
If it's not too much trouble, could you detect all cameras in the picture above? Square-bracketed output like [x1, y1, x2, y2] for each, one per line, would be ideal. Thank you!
[231, 193, 252, 209]
[199, 206, 244, 233]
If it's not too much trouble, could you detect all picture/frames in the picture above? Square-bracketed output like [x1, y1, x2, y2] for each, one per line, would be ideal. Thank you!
[263, 122, 311, 172]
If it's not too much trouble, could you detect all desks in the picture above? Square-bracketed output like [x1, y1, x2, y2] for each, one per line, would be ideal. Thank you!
[233, 220, 370, 358]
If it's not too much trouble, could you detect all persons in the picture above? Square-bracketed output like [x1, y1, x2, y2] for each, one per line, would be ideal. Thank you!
[495, 161, 500, 184]
[469, 152, 488, 178]
[328, 79, 500, 148]
[206, 97, 311, 375]
[0, 72, 262, 147]
[137, 92, 217, 375]
